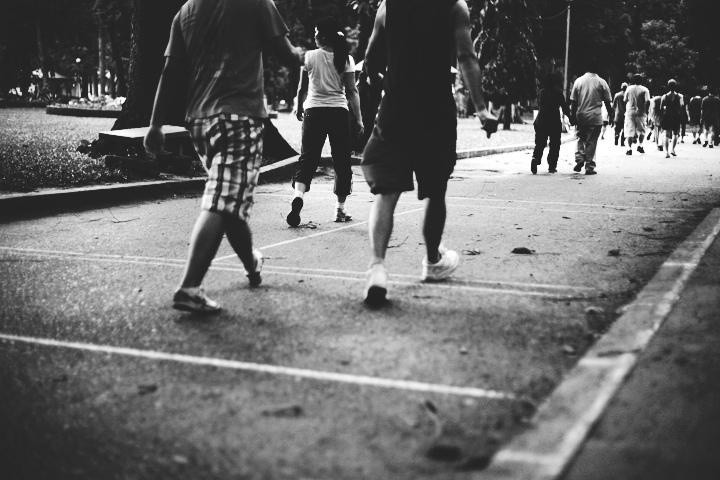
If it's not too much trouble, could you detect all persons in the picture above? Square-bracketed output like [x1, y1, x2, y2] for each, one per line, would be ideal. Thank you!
[362, 0, 499, 307]
[523, 62, 720, 176]
[288, 21, 366, 228]
[141, 0, 302, 313]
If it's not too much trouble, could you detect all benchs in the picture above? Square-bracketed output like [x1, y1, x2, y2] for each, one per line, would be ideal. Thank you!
[98, 110, 277, 157]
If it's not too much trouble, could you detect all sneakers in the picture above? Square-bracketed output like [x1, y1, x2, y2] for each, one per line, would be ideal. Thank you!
[362, 266, 388, 303]
[420, 249, 460, 281]
[586, 170, 597, 175]
[531, 158, 537, 174]
[333, 212, 352, 222]
[549, 168, 557, 173]
[245, 249, 264, 287]
[574, 160, 584, 172]
[285, 195, 303, 227]
[170, 286, 220, 313]
